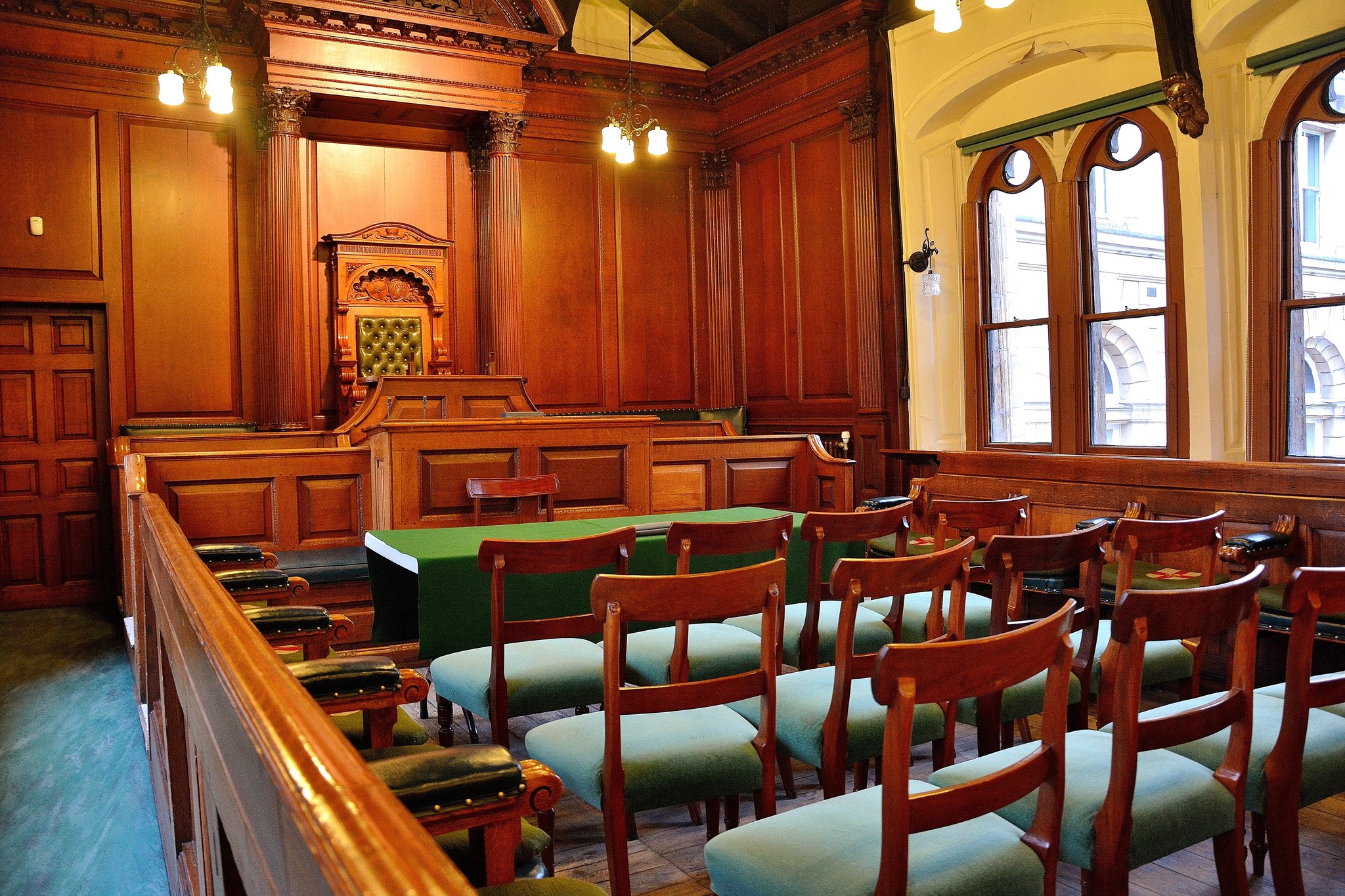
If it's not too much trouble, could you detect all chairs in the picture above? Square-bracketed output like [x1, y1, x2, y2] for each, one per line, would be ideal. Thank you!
[183, 463, 1345, 895]
[329, 298, 457, 435]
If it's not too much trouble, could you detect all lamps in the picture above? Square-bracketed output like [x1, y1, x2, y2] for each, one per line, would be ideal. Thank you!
[898, 225, 947, 299]
[600, 0, 674, 165]
[153, 1, 236, 116]
